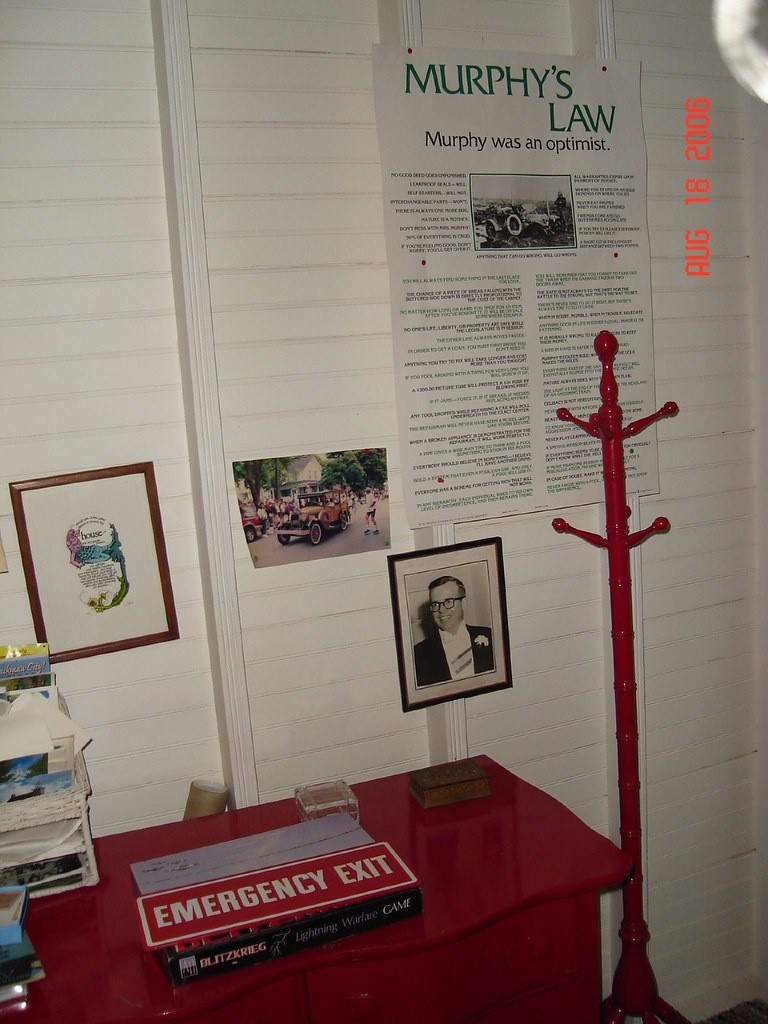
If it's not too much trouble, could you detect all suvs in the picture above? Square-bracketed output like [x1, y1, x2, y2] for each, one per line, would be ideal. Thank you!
[270, 485, 351, 546]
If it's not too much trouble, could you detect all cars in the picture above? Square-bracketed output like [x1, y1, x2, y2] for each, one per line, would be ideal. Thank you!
[484, 202, 560, 241]
[240, 504, 266, 543]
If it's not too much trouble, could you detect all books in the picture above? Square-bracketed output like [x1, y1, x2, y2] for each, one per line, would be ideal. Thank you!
[0, 886, 46, 1013]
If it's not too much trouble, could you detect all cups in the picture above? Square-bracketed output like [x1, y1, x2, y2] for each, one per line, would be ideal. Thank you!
[183, 780, 229, 822]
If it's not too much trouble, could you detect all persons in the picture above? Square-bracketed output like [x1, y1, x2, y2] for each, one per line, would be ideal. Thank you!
[248, 487, 389, 535]
[414, 576, 494, 687]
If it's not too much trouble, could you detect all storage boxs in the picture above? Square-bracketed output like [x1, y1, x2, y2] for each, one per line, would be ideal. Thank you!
[130, 811, 423, 985]
[0, 885, 44, 1002]
[408, 759, 492, 808]
[295, 780, 360, 823]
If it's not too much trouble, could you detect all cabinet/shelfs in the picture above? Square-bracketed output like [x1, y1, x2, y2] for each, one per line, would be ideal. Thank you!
[0, 753, 633, 1024]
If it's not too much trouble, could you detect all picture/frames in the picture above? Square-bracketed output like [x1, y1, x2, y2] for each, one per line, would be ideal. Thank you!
[9, 461, 180, 664]
[387, 537, 513, 713]
